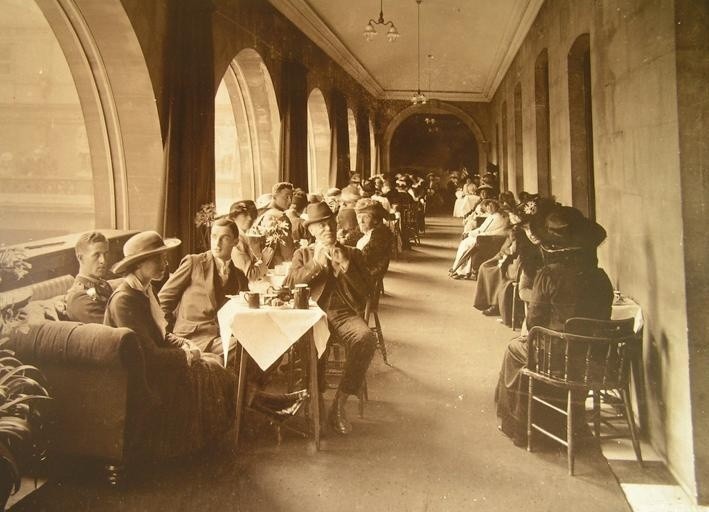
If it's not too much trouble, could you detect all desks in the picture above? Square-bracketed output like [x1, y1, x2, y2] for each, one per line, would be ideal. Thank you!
[452, 195, 481, 218]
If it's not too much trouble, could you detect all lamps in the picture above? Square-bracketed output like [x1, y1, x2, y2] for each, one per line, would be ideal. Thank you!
[423, 55, 437, 125]
[364, 0, 399, 45]
[410, 0, 427, 106]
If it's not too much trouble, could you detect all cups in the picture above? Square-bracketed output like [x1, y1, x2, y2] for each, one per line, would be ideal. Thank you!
[613, 291, 621, 306]
[293, 284, 312, 308]
[243, 291, 260, 310]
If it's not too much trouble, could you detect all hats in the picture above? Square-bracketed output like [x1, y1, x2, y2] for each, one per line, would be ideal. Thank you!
[476, 184, 492, 189]
[505, 196, 555, 229]
[481, 198, 499, 211]
[302, 202, 340, 230]
[355, 198, 383, 217]
[528, 206, 607, 254]
[112, 230, 182, 275]
[214, 200, 271, 222]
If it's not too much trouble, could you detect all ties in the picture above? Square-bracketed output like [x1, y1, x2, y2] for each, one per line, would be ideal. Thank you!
[220, 261, 228, 284]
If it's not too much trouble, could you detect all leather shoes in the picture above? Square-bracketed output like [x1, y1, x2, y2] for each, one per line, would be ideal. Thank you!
[328, 404, 353, 435]
[483, 304, 498, 315]
[453, 275, 469, 279]
[266, 389, 310, 428]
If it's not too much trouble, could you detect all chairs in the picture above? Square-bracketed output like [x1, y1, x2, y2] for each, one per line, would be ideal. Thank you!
[7, 190, 426, 485]
[471, 234, 646, 476]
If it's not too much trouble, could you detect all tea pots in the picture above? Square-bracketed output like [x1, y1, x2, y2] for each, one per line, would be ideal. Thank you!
[264, 283, 291, 307]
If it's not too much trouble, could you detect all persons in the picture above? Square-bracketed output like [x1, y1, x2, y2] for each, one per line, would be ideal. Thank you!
[496, 207, 614, 445]
[233, 182, 321, 282]
[65, 232, 306, 420]
[104, 230, 236, 463]
[157, 219, 283, 385]
[474, 192, 560, 333]
[324, 178, 400, 281]
[378, 174, 443, 249]
[448, 174, 514, 279]
[291, 202, 377, 433]
